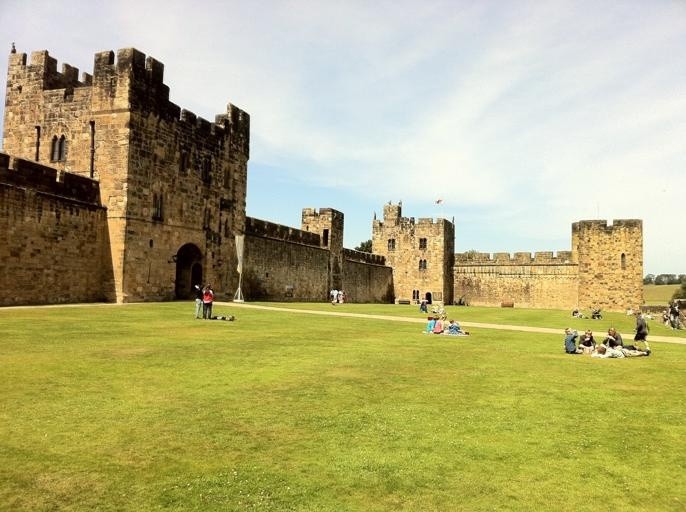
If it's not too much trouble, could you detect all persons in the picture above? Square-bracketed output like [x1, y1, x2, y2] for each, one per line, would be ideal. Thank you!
[338, 288, 344, 304]
[663, 301, 680, 329]
[427, 314, 469, 335]
[195, 282, 214, 319]
[331, 287, 338, 304]
[420, 301, 428, 313]
[564, 309, 651, 358]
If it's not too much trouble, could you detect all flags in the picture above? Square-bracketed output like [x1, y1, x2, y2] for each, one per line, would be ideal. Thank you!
[434, 198, 443, 205]
